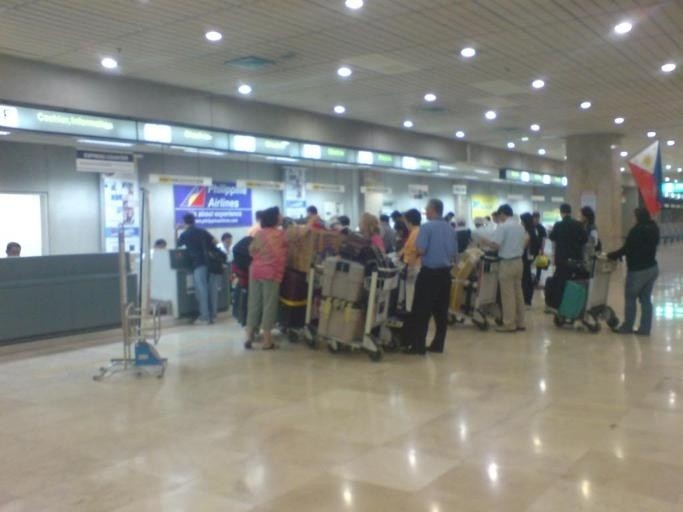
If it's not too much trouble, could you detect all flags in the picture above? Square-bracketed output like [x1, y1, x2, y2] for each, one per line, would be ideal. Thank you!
[627, 141, 663, 218]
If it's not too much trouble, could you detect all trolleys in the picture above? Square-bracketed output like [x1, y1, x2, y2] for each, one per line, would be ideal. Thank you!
[543, 240, 623, 333]
[282, 250, 545, 361]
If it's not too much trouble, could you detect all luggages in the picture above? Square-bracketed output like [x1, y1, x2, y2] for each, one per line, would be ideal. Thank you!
[230, 236, 264, 323]
[544, 254, 592, 320]
[450, 247, 496, 315]
[284, 224, 341, 273]
[317, 299, 391, 343]
[319, 252, 393, 306]
[276, 269, 315, 328]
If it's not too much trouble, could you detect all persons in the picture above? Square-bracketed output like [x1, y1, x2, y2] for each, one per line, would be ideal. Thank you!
[600, 207, 660, 335]
[6, 242, 21, 257]
[142, 239, 167, 260]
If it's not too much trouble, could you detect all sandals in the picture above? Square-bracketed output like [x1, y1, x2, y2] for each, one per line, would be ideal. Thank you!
[242, 341, 252, 349]
[261, 341, 281, 351]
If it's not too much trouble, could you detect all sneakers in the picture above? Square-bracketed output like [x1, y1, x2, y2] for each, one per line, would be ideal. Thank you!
[633, 327, 651, 335]
[403, 347, 424, 355]
[495, 321, 526, 333]
[427, 346, 442, 352]
[193, 315, 215, 327]
[611, 325, 631, 333]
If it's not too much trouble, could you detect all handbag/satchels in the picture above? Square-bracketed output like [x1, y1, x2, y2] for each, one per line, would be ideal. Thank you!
[169, 240, 194, 271]
[203, 247, 225, 274]
[535, 253, 550, 270]
